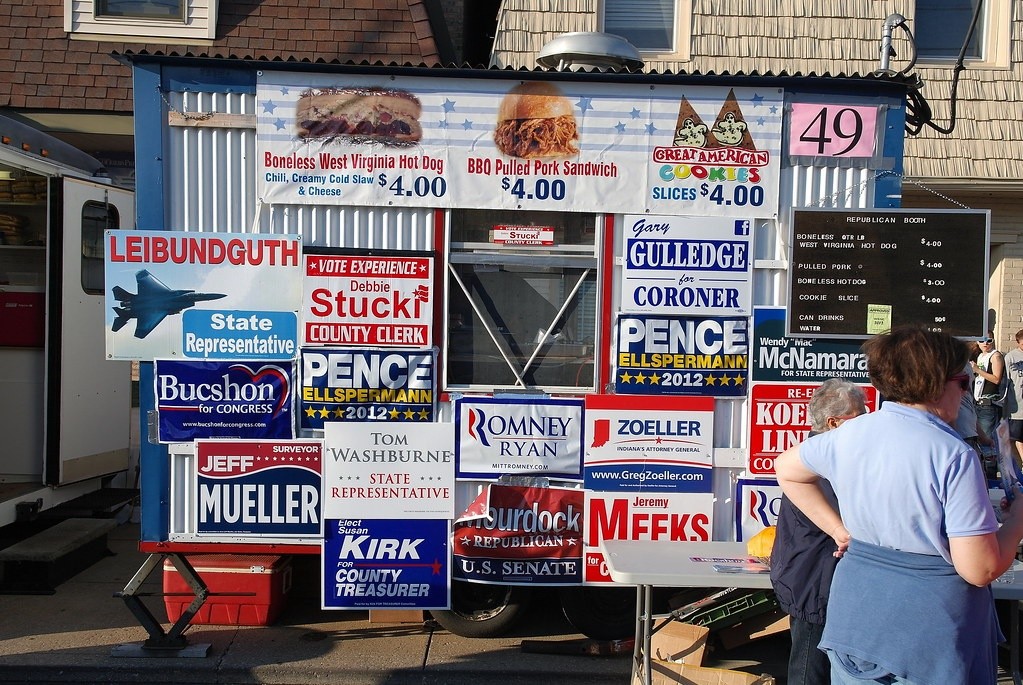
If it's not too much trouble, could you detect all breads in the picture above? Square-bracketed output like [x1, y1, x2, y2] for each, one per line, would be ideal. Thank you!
[0, 178, 52, 245]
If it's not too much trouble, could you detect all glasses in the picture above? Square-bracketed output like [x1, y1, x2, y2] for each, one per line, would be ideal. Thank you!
[944, 373, 970, 392]
[978, 338, 995, 344]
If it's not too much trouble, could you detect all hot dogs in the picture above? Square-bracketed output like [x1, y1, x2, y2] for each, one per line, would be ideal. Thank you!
[294, 88, 425, 144]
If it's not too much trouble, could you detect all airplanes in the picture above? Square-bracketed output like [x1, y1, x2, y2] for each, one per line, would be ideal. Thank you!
[111, 270, 227, 339]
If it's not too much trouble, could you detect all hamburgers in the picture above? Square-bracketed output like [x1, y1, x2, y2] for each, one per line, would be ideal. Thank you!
[493, 83, 581, 159]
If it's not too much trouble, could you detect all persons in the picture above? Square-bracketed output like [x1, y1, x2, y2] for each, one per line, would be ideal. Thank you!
[770, 380, 866, 685]
[956, 333, 1023, 495]
[774, 326, 1023, 685]
